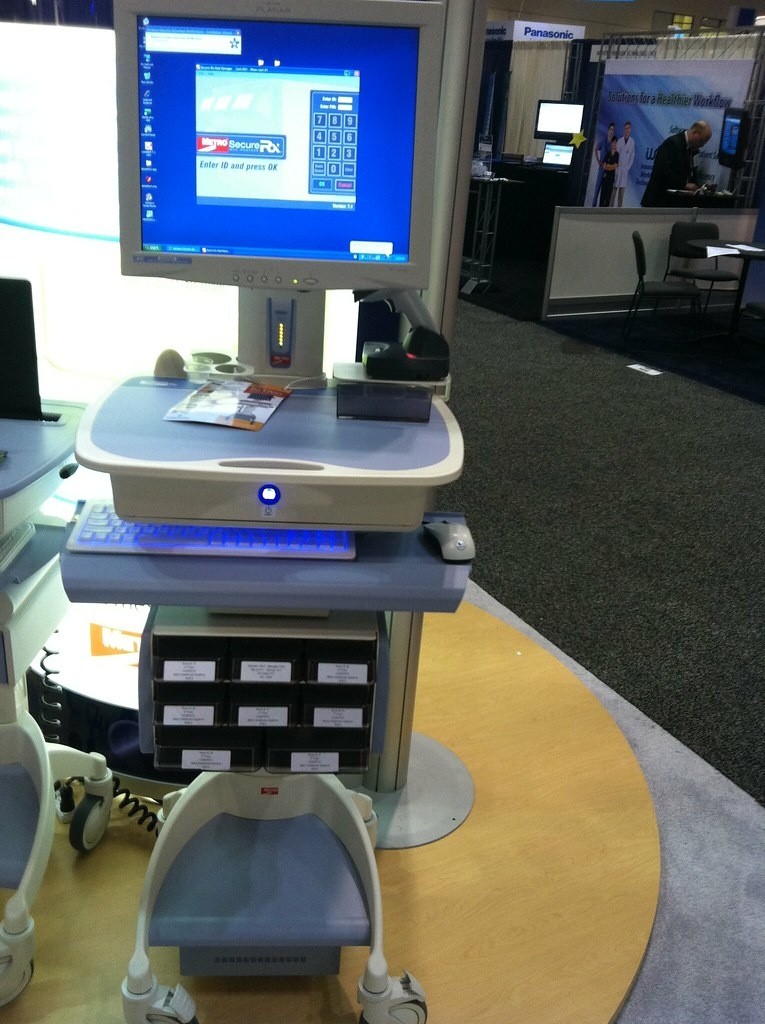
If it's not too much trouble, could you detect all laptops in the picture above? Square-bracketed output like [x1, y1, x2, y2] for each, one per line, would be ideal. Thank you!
[531, 141, 575, 172]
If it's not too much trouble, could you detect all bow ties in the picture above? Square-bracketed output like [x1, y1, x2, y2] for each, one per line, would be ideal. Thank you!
[688, 147, 697, 157]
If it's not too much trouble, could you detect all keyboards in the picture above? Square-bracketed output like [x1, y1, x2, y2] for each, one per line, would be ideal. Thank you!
[64, 498, 357, 560]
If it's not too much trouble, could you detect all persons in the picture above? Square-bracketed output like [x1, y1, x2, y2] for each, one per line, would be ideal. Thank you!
[640, 121, 712, 207]
[592, 122, 634, 207]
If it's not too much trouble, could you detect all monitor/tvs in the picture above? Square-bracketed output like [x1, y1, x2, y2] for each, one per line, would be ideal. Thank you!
[533, 98, 585, 142]
[719, 108, 750, 170]
[114, 0, 449, 391]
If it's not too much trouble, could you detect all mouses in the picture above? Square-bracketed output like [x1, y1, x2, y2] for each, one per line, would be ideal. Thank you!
[422, 517, 477, 561]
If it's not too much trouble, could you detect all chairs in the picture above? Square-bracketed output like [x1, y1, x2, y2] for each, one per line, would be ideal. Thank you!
[629, 230, 704, 340]
[652, 222, 741, 320]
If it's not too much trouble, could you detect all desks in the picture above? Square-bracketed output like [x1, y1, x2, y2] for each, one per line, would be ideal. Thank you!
[687, 239, 765, 334]
